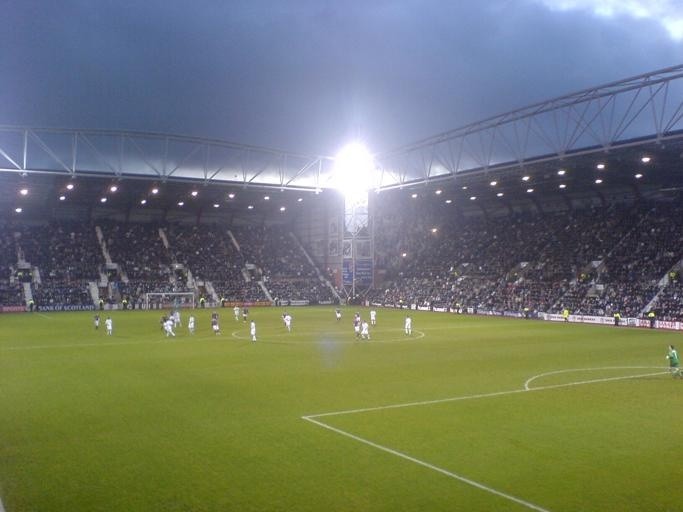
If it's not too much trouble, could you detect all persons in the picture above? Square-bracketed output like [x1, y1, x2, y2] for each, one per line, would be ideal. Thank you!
[232, 304, 292, 341]
[666, 345, 683, 379]
[94, 313, 113, 335]
[335, 305, 413, 340]
[353, 190, 681, 329]
[1, 189, 340, 311]
[160, 309, 220, 337]
